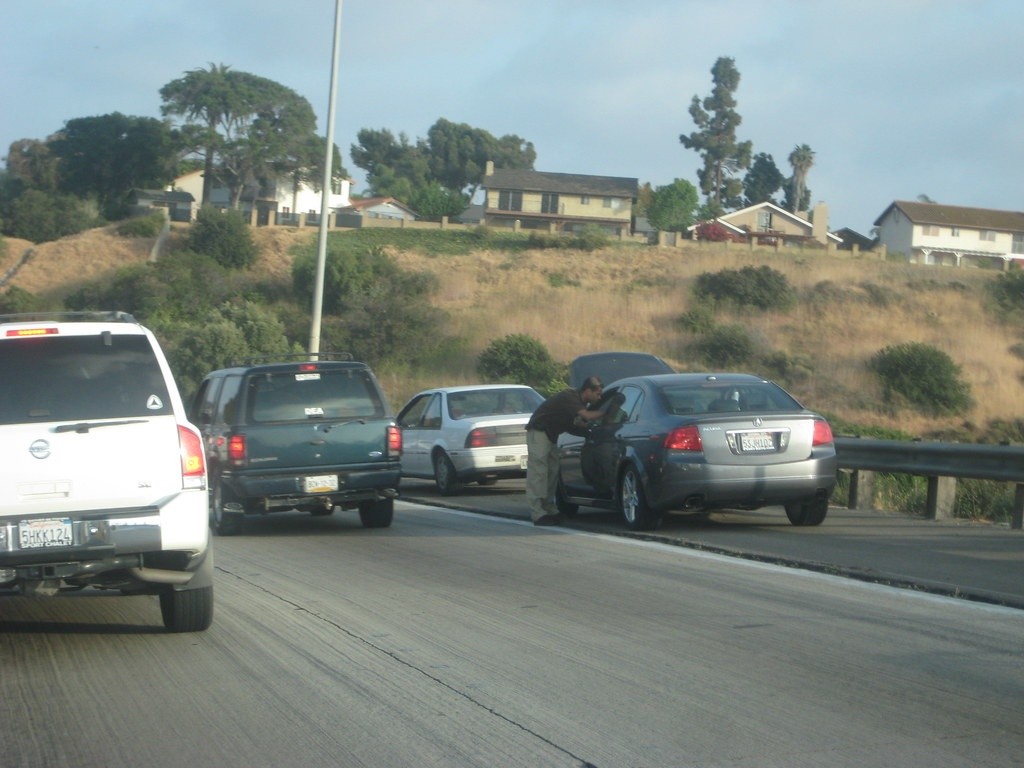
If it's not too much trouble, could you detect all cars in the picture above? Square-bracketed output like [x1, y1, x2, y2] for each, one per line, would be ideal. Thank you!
[394, 384, 546, 497]
[551, 351, 838, 532]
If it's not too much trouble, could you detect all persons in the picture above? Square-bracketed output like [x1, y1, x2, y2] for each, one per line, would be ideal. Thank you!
[524, 377, 606, 526]
[606, 392, 626, 426]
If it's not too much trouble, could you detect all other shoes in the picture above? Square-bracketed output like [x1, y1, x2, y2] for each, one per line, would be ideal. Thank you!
[533, 512, 567, 527]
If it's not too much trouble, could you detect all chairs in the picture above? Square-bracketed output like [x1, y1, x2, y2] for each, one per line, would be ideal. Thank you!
[744, 393, 769, 410]
[709, 399, 740, 413]
[667, 395, 697, 414]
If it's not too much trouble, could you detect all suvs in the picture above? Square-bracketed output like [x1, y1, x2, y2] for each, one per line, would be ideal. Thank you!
[181, 351, 405, 538]
[0, 308, 215, 632]
